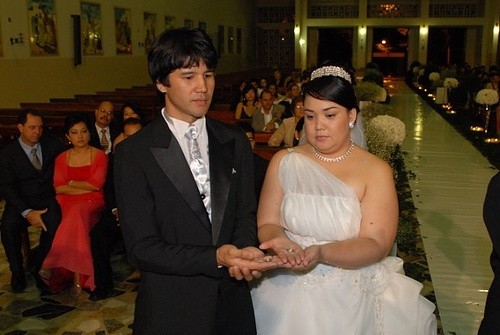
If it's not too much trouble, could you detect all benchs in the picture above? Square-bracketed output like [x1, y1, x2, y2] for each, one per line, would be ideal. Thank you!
[0, 63, 297, 270]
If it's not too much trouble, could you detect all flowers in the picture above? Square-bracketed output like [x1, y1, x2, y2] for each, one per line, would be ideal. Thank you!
[355, 59, 410, 184]
[410, 62, 499, 132]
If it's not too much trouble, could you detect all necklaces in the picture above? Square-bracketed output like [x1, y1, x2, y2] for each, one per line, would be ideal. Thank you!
[311, 139, 356, 164]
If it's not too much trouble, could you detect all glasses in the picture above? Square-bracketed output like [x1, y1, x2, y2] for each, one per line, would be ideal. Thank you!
[248, 137, 254, 141]
[99, 107, 112, 116]
[296, 105, 303, 109]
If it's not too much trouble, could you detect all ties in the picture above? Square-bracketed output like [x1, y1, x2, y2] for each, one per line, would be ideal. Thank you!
[31, 148, 42, 170]
[184, 124, 212, 225]
[100, 129, 109, 152]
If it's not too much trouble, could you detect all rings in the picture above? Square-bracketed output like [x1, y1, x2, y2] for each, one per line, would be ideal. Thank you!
[287, 248, 293, 252]
[264, 256, 273, 262]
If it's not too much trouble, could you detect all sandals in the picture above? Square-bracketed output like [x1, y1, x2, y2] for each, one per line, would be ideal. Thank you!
[38, 269, 54, 281]
[70, 279, 81, 298]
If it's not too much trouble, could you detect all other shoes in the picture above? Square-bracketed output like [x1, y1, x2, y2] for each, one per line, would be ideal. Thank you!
[89, 287, 113, 300]
[11, 270, 27, 293]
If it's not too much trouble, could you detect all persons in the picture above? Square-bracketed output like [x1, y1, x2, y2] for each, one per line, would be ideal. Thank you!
[477, 172, 500, 335]
[362, 62, 384, 87]
[0, 108, 61, 292]
[405, 60, 500, 92]
[88, 100, 146, 155]
[232, 69, 316, 150]
[247, 58, 438, 335]
[39, 112, 142, 304]
[111, 28, 278, 335]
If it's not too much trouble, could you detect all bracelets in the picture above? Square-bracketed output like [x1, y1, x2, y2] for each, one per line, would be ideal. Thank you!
[69, 180, 72, 185]
